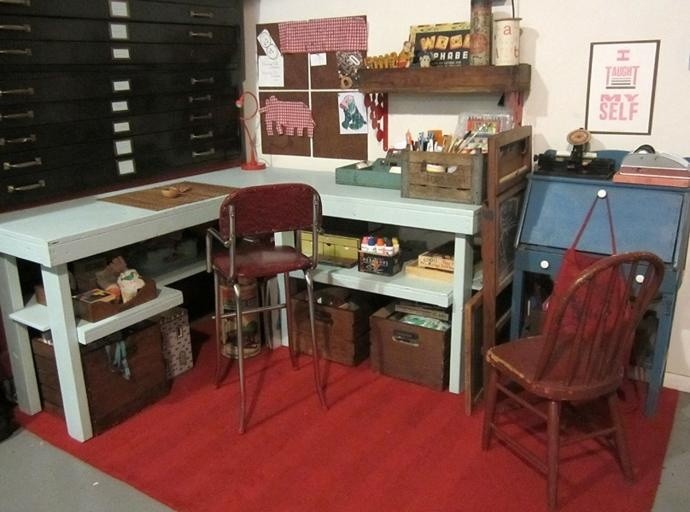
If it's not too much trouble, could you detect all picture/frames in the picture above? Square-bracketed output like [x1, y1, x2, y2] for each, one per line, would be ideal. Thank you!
[586, 38, 661, 137]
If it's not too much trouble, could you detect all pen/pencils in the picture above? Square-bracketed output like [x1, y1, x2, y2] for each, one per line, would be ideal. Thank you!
[363, 52, 397, 70]
[406, 124, 486, 154]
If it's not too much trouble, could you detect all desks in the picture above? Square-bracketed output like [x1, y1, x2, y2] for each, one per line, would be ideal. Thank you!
[0, 167, 483, 444]
[512, 143, 689, 423]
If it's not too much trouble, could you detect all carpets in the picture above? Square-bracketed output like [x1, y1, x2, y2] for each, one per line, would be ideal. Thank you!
[13, 303, 678, 511]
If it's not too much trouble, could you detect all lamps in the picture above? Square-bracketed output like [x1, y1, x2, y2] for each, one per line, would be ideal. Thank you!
[235, 91, 265, 172]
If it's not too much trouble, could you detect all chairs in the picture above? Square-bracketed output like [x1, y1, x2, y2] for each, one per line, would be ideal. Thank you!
[204, 183, 332, 433]
[481, 253, 664, 511]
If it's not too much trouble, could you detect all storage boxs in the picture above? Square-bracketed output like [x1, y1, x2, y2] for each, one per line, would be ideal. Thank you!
[368, 296, 451, 393]
[150, 302, 193, 379]
[400, 144, 488, 202]
[287, 280, 368, 369]
[356, 250, 403, 279]
[33, 327, 167, 433]
[300, 221, 398, 265]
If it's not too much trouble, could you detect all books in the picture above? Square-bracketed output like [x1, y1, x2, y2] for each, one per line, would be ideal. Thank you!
[394, 303, 451, 321]
[400, 297, 451, 312]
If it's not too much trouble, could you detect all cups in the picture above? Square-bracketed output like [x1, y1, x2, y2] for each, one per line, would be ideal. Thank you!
[493, 18, 521, 67]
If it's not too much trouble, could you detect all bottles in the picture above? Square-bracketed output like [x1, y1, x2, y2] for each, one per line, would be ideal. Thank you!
[359, 236, 399, 255]
